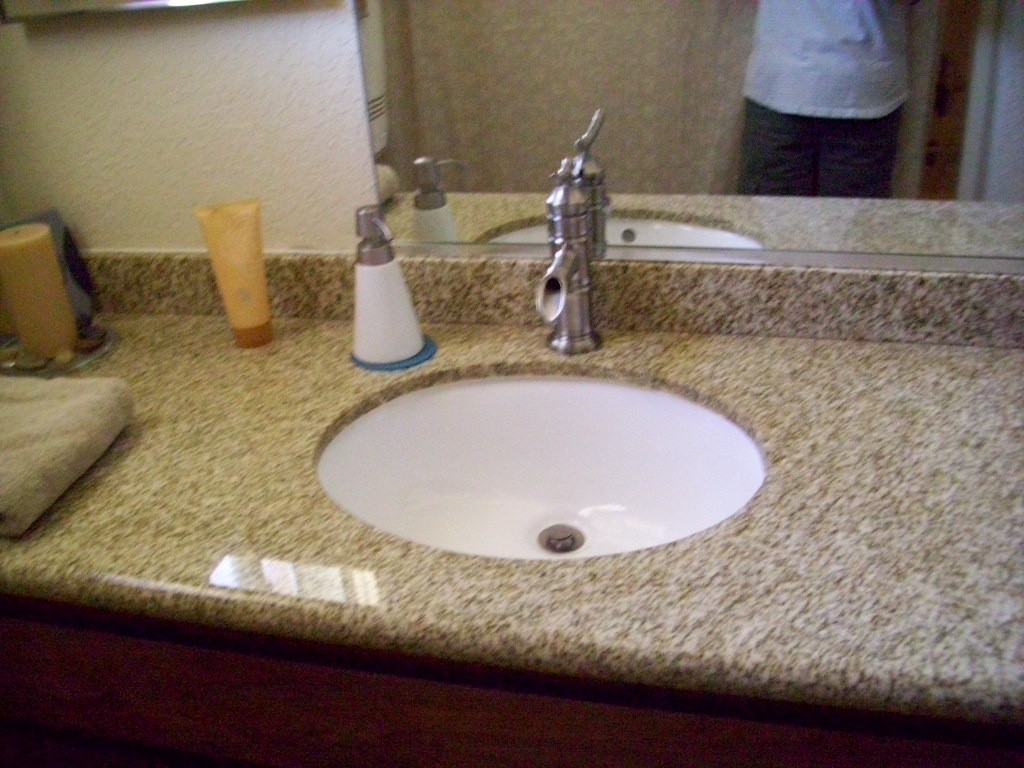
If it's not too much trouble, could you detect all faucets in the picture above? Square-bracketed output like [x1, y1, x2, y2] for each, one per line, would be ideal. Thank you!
[530, 153, 604, 353]
[570, 100, 608, 249]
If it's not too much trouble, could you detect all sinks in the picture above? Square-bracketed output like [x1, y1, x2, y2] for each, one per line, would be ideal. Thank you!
[309, 363, 768, 561]
[472, 207, 767, 254]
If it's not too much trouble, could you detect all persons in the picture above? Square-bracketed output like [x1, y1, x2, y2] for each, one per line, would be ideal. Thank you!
[734, 0, 912, 198]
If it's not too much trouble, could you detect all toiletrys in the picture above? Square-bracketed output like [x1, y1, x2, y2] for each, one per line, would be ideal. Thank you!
[351, 206, 425, 364]
[193, 200, 276, 348]
[410, 157, 470, 244]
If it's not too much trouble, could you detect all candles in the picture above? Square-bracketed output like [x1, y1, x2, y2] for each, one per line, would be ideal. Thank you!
[0, 222, 80, 357]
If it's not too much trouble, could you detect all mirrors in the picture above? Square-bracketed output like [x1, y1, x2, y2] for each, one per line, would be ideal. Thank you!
[352, 0, 1024, 276]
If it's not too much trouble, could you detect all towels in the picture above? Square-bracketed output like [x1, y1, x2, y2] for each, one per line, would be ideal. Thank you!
[373, 163, 400, 203]
[3, 373, 133, 536]
[354, 0, 387, 157]
